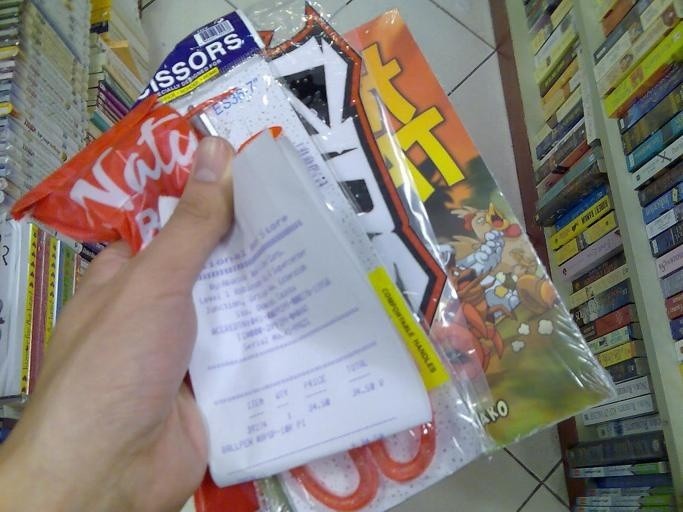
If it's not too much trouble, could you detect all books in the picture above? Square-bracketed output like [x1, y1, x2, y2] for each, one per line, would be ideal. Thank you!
[91, 1, 151, 148]
[1, 0, 91, 253]
[0, 220, 95, 409]
[521, 0, 682, 509]
